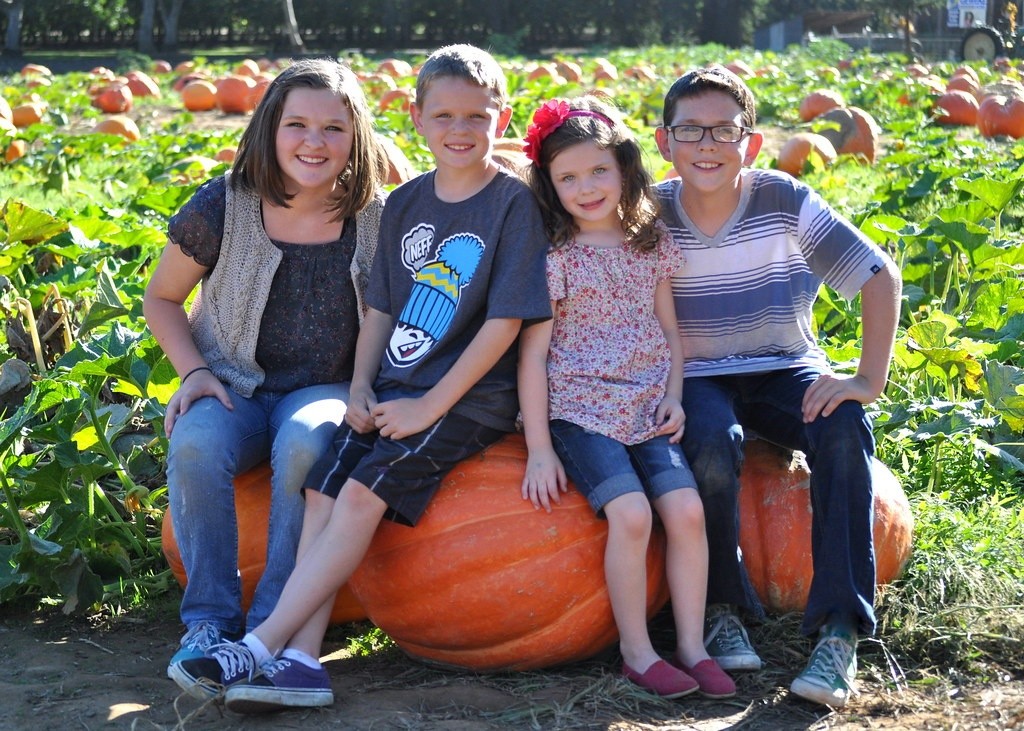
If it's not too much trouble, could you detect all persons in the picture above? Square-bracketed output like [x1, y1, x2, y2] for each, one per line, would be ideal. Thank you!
[171, 45, 552, 709]
[648, 67, 903, 706]
[142, 58, 386, 678]
[518, 93, 740, 698]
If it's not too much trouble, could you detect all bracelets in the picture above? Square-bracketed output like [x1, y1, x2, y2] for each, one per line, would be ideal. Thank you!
[181, 366, 213, 384]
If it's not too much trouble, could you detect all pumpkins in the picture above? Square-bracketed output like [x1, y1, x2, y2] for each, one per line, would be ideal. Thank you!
[162, 464, 366, 625]
[347, 430, 671, 673]
[739, 437, 913, 616]
[0, 58, 1024, 183]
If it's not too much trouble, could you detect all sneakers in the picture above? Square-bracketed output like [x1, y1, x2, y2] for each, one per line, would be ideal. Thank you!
[167, 621, 234, 679]
[225, 648, 334, 714]
[789, 623, 858, 708]
[171, 638, 261, 703]
[704, 603, 761, 670]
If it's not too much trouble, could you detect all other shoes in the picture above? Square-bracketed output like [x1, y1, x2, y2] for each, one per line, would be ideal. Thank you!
[622, 661, 699, 698]
[672, 655, 736, 699]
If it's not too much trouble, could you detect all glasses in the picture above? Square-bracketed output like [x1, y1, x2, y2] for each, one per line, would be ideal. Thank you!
[666, 124, 753, 143]
